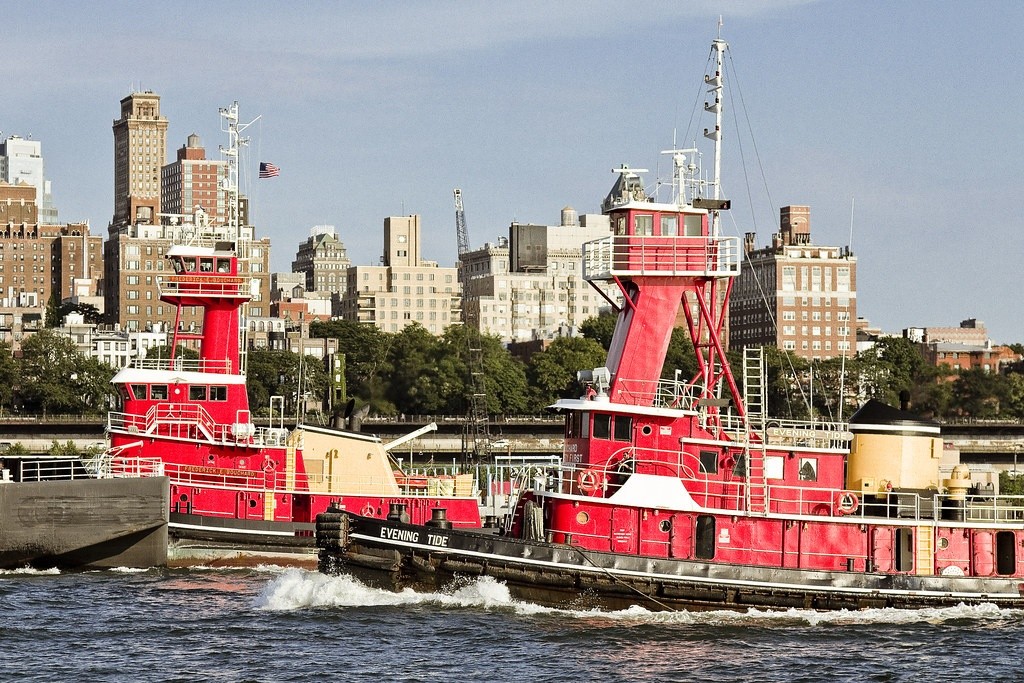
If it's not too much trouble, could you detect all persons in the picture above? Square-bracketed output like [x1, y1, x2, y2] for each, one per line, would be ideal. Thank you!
[617, 451, 636, 485]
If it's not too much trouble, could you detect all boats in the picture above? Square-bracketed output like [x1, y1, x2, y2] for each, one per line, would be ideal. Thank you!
[81, 13, 1024, 618]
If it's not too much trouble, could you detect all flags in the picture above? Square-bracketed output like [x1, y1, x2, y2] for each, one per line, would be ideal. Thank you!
[259, 163, 281, 178]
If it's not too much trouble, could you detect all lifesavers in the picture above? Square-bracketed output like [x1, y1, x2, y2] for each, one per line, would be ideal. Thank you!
[190, 425, 204, 438]
[836, 492, 860, 515]
[577, 468, 600, 492]
[261, 458, 277, 472]
